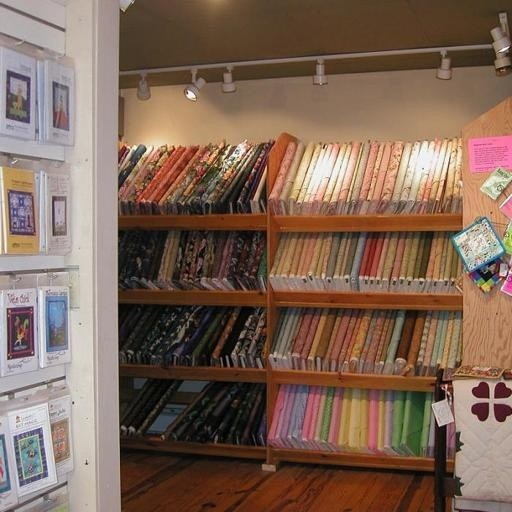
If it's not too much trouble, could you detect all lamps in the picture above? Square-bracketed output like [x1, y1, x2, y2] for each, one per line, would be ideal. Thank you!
[119, 12, 512, 103]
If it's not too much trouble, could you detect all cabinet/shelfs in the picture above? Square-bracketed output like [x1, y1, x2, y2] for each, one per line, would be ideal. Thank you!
[118, 132, 461, 474]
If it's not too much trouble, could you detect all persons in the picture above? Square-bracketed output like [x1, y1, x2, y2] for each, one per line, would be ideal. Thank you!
[11, 197, 32, 230]
[55, 94, 68, 130]
[12, 87, 26, 113]
